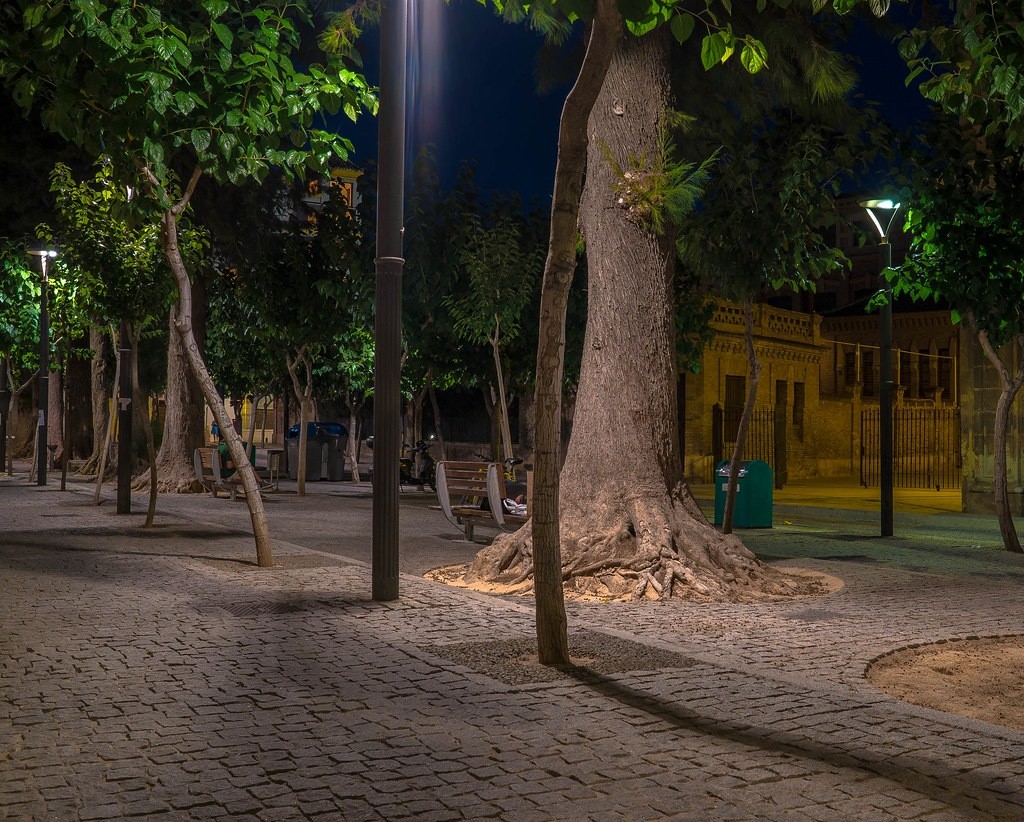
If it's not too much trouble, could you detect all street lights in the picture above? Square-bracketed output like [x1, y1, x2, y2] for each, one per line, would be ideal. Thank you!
[26, 239, 62, 486]
[858, 191, 909, 536]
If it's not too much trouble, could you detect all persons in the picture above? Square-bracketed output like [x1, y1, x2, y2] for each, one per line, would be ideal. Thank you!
[211, 419, 219, 442]
[218, 435, 275, 498]
[232, 415, 240, 433]
[480, 458, 528, 515]
[419, 447, 436, 479]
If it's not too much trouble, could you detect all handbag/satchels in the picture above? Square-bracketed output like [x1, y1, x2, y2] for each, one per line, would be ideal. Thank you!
[503, 498, 527, 516]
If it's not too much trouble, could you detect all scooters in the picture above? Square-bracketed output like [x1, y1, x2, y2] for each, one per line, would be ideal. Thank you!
[366, 434, 449, 492]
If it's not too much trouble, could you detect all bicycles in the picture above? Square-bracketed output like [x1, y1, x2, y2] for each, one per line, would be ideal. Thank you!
[457, 449, 523, 541]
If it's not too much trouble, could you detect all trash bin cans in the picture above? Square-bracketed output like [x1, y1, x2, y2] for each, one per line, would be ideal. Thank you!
[218, 441, 256, 477]
[284, 421, 349, 481]
[713, 458, 774, 528]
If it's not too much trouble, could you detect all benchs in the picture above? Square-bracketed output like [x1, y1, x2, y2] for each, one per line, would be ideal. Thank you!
[434, 460, 531, 541]
[193, 448, 245, 501]
